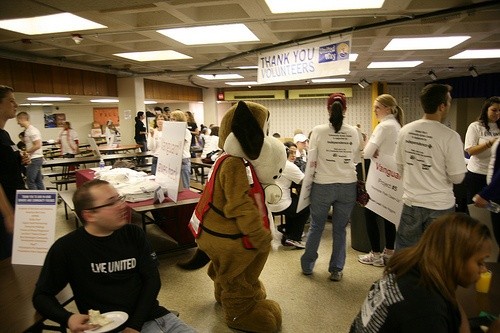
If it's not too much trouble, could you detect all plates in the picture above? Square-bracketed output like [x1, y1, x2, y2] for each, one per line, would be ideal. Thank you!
[82, 311, 129, 333]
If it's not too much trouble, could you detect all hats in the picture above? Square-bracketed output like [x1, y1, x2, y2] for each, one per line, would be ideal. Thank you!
[294, 134, 307, 144]
[328, 94, 347, 109]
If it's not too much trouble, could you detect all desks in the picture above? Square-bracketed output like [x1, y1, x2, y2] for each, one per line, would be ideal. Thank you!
[0, 256, 75, 333]
[40, 140, 244, 255]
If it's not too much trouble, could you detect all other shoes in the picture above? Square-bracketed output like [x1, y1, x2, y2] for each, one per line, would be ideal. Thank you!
[285, 232, 306, 248]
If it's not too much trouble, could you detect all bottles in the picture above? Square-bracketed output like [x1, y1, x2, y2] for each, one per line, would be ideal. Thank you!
[99, 158, 104, 171]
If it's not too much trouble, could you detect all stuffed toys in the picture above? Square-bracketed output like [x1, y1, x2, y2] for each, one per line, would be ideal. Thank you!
[187, 98, 288, 333]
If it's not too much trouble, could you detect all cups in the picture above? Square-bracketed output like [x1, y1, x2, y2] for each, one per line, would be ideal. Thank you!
[475, 270, 492, 294]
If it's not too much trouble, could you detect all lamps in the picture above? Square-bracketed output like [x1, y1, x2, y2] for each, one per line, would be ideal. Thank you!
[468, 65, 478, 77]
[428, 67, 439, 80]
[358, 77, 372, 89]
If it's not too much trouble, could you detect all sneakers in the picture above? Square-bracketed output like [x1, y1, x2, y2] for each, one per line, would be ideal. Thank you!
[330, 270, 343, 281]
[303, 270, 313, 274]
[358, 251, 393, 267]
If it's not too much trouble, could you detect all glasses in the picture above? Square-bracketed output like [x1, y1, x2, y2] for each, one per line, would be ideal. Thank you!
[87, 195, 126, 210]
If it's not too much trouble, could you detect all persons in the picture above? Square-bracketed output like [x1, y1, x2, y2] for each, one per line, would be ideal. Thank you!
[357, 92, 410, 268]
[134, 111, 148, 165]
[0, 84, 33, 258]
[16, 141, 44, 183]
[391, 83, 468, 282]
[149, 113, 169, 181]
[261, 129, 368, 250]
[16, 110, 46, 191]
[455, 94, 500, 219]
[348, 212, 494, 333]
[149, 103, 224, 163]
[300, 93, 364, 282]
[31, 178, 205, 333]
[169, 110, 193, 192]
[18, 130, 44, 164]
[54, 121, 80, 180]
[486, 131, 500, 267]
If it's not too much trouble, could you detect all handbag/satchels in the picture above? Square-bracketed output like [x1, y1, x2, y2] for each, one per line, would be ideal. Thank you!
[357, 179, 369, 205]
